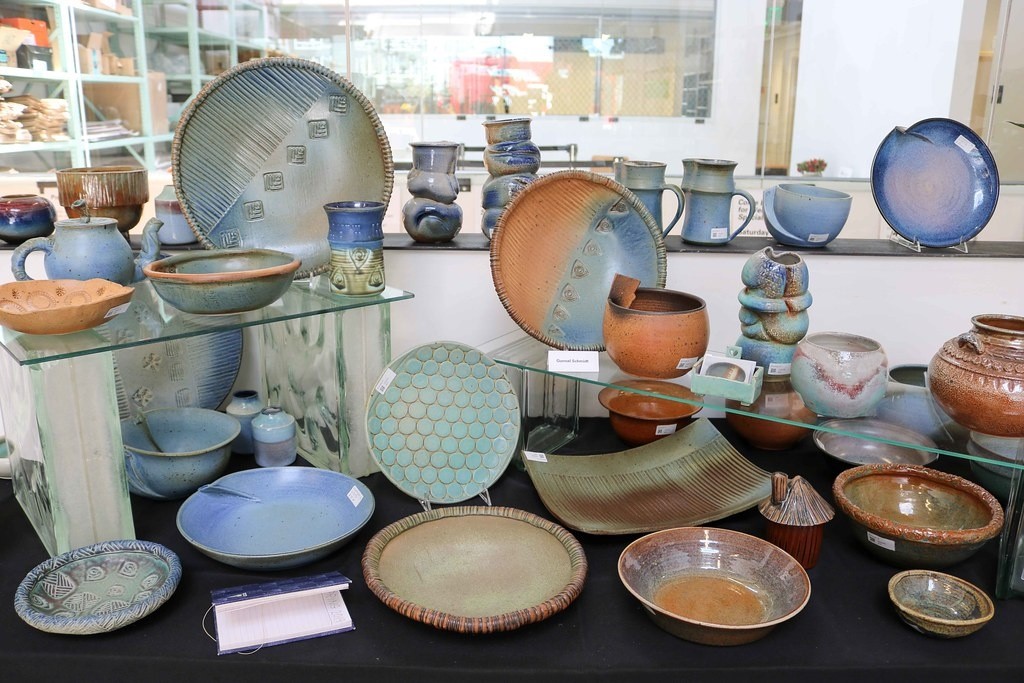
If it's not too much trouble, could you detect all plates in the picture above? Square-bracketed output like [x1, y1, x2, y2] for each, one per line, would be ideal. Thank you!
[111, 330, 244, 418]
[172, 56, 394, 278]
[870, 117, 1000, 248]
[365, 341, 792, 632]
[490, 172, 666, 351]
[14, 542, 181, 635]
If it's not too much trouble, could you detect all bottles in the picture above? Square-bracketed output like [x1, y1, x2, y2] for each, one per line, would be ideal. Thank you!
[734, 247, 812, 382]
[928, 315, 1024, 437]
[400, 117, 542, 242]
[0, 195, 56, 245]
[251, 406, 298, 468]
[226, 390, 263, 457]
[154, 184, 196, 245]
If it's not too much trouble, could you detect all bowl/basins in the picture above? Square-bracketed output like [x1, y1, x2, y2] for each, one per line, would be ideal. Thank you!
[792, 332, 1024, 638]
[597, 379, 705, 439]
[175, 467, 375, 565]
[618, 523, 812, 646]
[0, 278, 135, 333]
[142, 248, 302, 314]
[602, 271, 709, 378]
[56, 165, 149, 230]
[119, 405, 241, 497]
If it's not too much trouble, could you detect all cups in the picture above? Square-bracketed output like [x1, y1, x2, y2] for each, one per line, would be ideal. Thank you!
[613, 156, 756, 246]
[763, 183, 853, 248]
[322, 200, 387, 296]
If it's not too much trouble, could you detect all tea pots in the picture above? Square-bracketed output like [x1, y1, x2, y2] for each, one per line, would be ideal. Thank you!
[10, 200, 164, 284]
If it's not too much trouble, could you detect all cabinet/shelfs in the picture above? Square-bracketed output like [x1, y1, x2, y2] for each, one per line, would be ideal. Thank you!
[0, 0, 268, 178]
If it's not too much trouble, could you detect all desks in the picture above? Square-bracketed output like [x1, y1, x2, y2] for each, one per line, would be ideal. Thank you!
[0, 418, 1024, 683]
